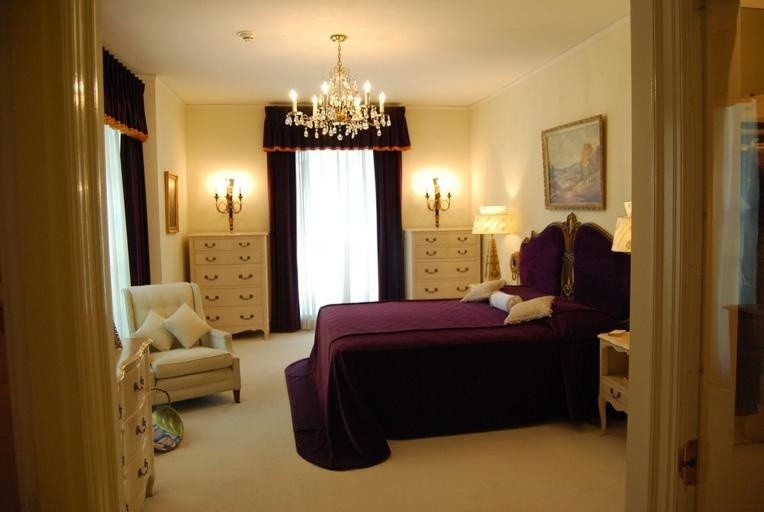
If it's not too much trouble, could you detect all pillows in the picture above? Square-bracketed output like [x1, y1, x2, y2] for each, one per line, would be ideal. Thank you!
[163, 302, 212, 348]
[489, 290, 522, 313]
[504, 295, 554, 325]
[460, 280, 506, 302]
[132, 309, 177, 351]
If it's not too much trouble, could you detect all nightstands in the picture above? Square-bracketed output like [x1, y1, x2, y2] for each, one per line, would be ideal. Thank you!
[597, 332, 629, 432]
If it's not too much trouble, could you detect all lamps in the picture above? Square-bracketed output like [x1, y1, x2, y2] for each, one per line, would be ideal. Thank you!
[471, 206, 517, 280]
[285, 35, 391, 140]
[610, 202, 632, 252]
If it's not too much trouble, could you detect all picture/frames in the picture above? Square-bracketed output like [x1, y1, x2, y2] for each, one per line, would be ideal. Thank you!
[164, 171, 180, 233]
[541, 114, 608, 211]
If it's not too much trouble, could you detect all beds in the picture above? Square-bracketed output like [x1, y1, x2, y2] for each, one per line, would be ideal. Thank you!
[284, 212, 631, 472]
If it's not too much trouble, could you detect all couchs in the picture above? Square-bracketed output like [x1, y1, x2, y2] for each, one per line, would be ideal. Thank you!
[120, 282, 242, 412]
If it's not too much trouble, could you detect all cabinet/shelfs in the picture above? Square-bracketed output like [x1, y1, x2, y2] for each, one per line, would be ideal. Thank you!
[402, 226, 481, 300]
[115, 337, 157, 512]
[188, 231, 269, 340]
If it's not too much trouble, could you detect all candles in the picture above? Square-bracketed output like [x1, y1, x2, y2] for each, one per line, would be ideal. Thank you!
[215, 186, 217, 194]
[426, 183, 427, 192]
[225, 179, 227, 195]
[238, 186, 241, 196]
